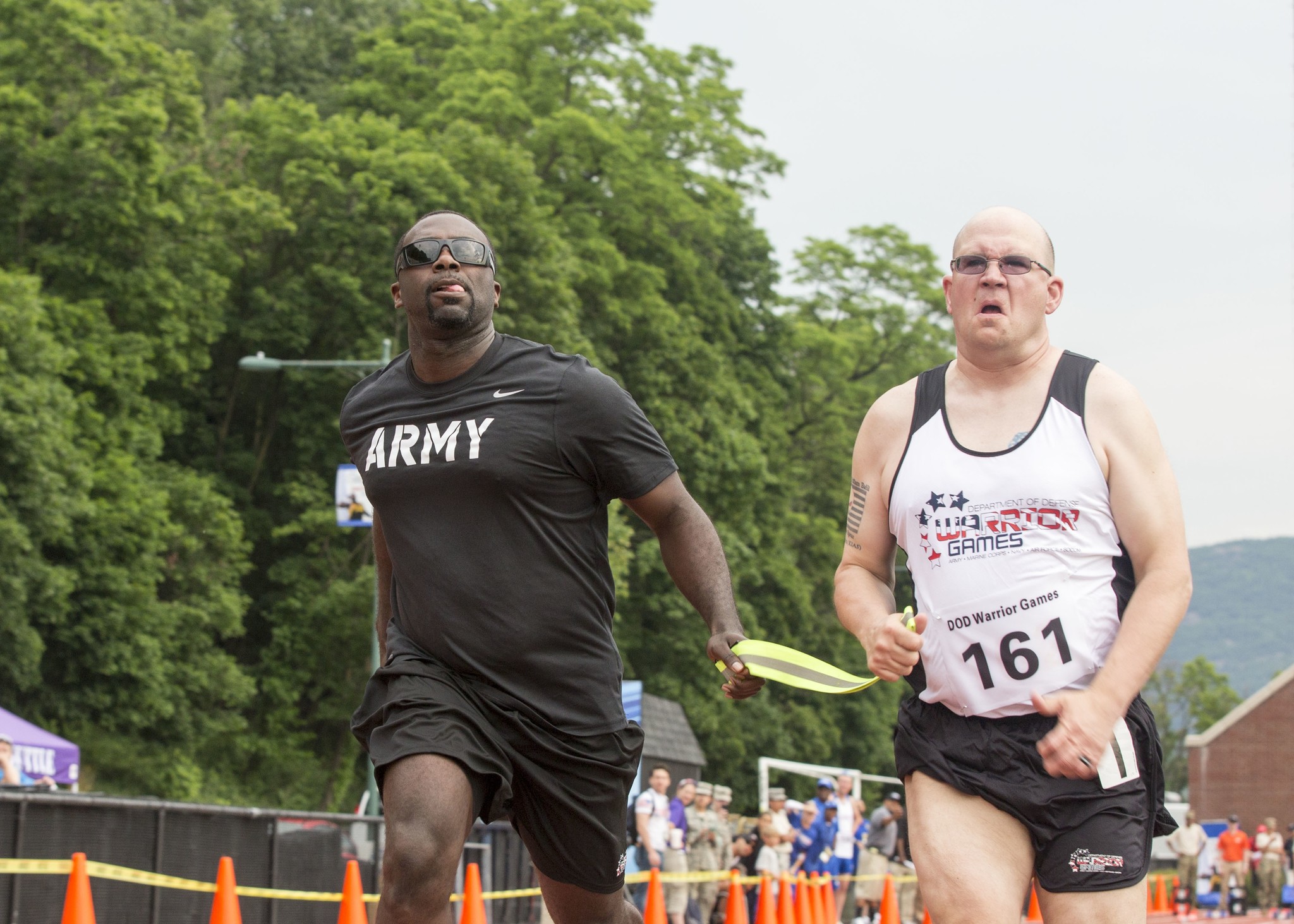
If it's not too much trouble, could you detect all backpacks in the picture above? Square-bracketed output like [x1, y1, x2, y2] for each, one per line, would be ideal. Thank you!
[626, 790, 654, 844]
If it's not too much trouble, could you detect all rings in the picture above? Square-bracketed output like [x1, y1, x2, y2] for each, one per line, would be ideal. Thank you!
[1080, 755, 1090, 765]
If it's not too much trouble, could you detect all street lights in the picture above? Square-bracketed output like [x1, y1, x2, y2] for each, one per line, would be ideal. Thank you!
[239, 338, 393, 821]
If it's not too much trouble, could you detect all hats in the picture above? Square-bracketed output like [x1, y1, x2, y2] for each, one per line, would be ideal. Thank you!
[714, 784, 733, 802]
[824, 802, 839, 810]
[818, 778, 837, 791]
[696, 781, 714, 796]
[884, 790, 902, 803]
[769, 787, 788, 802]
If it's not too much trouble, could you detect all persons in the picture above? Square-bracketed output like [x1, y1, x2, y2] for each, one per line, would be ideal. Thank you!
[831, 206, 1197, 924]
[1208, 814, 1294, 918]
[341, 209, 766, 924]
[1167, 808, 1207, 915]
[627, 763, 909, 924]
[0, 734, 57, 787]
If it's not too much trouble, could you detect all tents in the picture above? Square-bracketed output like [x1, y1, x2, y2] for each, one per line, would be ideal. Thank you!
[0, 708, 80, 788]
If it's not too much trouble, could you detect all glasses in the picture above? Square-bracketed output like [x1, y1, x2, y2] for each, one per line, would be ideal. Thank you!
[744, 838, 755, 851]
[395, 237, 494, 280]
[949, 255, 1051, 276]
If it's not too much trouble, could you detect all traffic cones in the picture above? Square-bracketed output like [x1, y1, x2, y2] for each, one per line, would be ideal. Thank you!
[1170, 875, 1180, 914]
[335, 859, 371, 924]
[754, 872, 779, 924]
[724, 868, 750, 924]
[210, 857, 244, 924]
[1146, 876, 1157, 915]
[1153, 873, 1173, 915]
[808, 870, 826, 924]
[878, 872, 902, 924]
[821, 871, 838, 924]
[1024, 881, 1046, 924]
[794, 870, 811, 924]
[642, 868, 670, 924]
[60, 851, 99, 924]
[459, 862, 489, 924]
[921, 905, 934, 924]
[776, 870, 796, 924]
